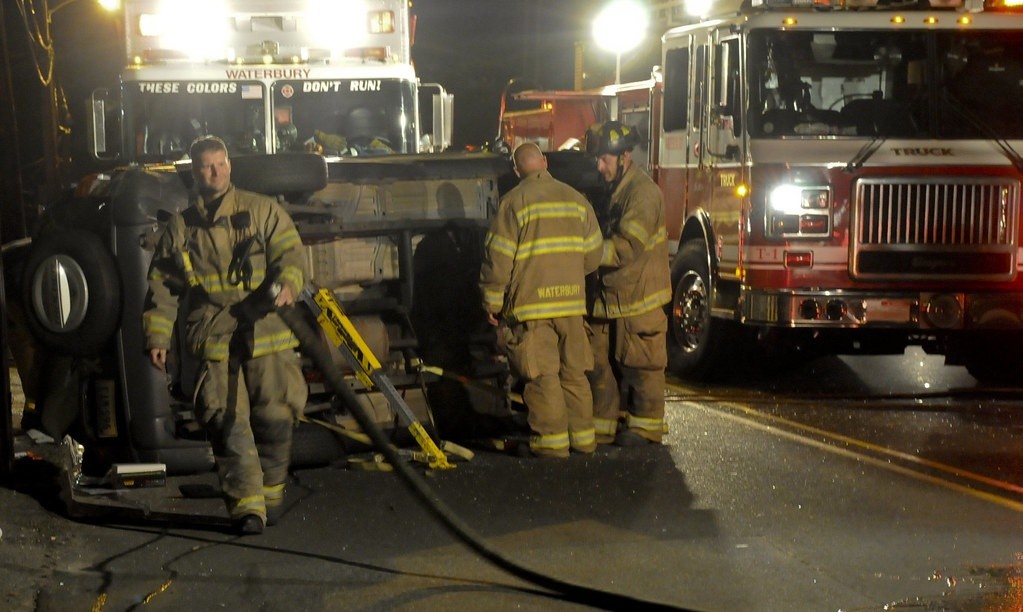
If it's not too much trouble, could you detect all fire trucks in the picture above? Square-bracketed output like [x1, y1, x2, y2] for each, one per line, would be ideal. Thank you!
[494, 0, 1022, 400]
[81, 1, 456, 171]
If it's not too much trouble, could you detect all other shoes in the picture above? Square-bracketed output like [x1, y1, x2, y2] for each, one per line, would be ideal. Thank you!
[239, 514, 262, 534]
[617, 430, 650, 446]
[505, 437, 558, 459]
[266, 508, 277, 524]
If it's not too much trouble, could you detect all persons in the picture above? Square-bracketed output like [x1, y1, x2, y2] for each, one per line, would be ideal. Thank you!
[585, 120, 671, 448]
[139, 134, 311, 535]
[312, 100, 398, 156]
[909, 32, 1016, 130]
[2, 178, 66, 437]
[478, 142, 604, 459]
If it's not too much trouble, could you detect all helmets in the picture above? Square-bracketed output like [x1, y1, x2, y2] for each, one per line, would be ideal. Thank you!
[582, 121, 640, 158]
